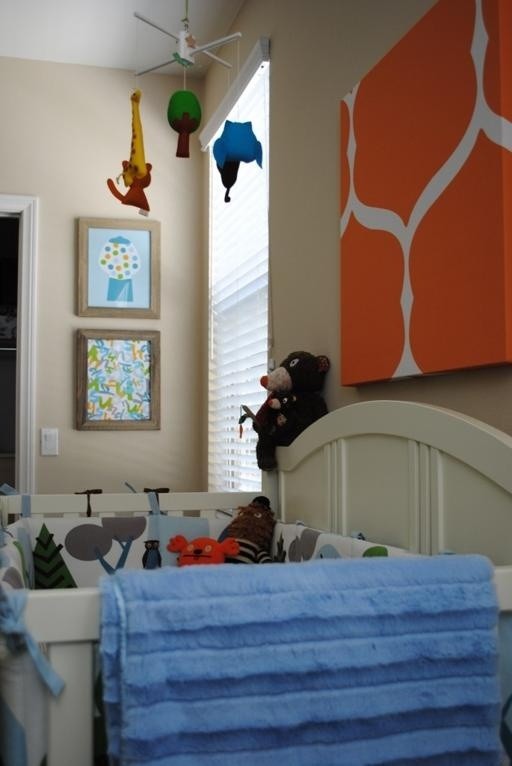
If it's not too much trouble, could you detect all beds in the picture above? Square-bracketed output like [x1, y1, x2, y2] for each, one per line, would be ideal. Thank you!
[0, 398, 511, 766]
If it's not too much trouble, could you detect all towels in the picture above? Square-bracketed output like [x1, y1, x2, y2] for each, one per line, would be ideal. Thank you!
[96, 551, 508, 766]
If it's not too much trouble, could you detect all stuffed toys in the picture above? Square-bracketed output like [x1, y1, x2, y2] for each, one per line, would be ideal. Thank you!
[213, 121, 262, 202]
[107, 89, 152, 217]
[167, 535, 239, 566]
[218, 495, 276, 564]
[238, 349, 331, 472]
[167, 90, 201, 159]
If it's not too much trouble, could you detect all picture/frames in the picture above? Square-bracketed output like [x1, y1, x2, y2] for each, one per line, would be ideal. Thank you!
[76, 329, 162, 432]
[76, 216, 161, 320]
